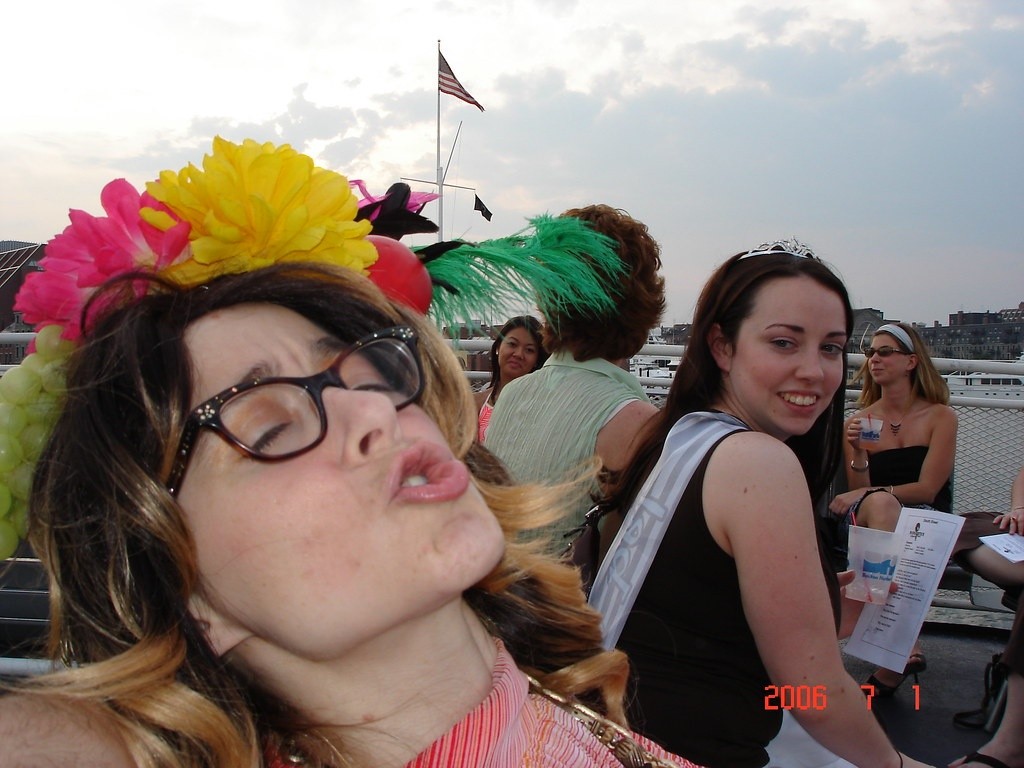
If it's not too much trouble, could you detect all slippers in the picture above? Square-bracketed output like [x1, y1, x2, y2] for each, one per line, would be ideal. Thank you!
[964, 750, 1010, 768]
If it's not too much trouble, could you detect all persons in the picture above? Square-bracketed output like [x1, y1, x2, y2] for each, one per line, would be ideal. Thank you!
[943, 465, 1024, 768]
[470, 316, 548, 448]
[485, 206, 665, 597]
[591, 239, 938, 768]
[0, 263, 703, 768]
[829, 323, 959, 531]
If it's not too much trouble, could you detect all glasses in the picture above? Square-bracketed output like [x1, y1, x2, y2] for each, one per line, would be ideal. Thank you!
[862, 344, 910, 357]
[157, 322, 427, 520]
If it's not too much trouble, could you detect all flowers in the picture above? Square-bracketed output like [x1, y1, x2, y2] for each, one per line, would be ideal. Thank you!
[13, 136, 378, 357]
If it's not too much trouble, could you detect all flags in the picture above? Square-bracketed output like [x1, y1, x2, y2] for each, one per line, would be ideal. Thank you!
[438, 52, 485, 112]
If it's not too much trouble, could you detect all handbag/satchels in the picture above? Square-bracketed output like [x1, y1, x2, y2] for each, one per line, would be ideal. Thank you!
[552, 481, 628, 599]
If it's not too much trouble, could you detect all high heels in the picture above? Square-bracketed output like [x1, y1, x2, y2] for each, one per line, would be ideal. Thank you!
[898, 653, 928, 685]
[865, 670, 908, 700]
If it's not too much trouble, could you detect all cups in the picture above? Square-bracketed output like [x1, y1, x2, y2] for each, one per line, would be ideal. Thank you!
[859, 417, 884, 444]
[844, 525, 904, 606]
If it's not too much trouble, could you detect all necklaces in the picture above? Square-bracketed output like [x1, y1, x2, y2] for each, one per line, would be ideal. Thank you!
[885, 415, 904, 436]
[283, 672, 681, 768]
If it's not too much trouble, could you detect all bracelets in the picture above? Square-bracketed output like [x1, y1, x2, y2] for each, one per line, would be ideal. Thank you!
[894, 747, 903, 768]
[890, 485, 894, 494]
[1015, 506, 1024, 509]
[850, 460, 869, 471]
